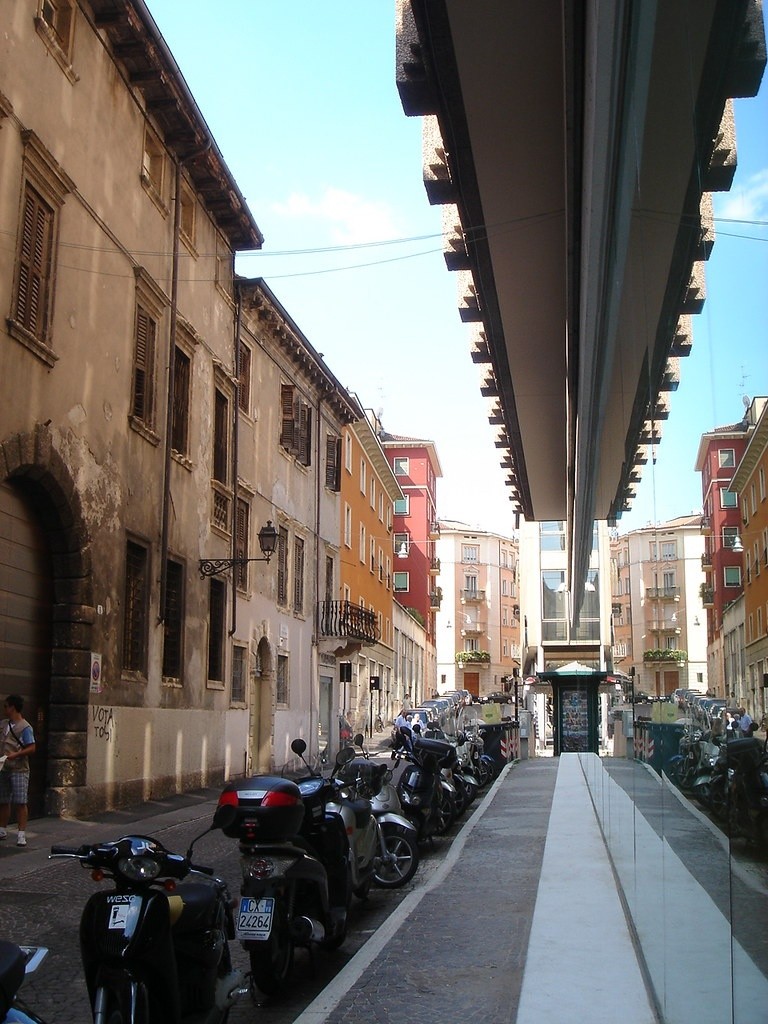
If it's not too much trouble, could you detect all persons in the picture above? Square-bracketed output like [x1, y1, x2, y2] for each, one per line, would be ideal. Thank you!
[0, 694, 35, 846]
[725, 692, 754, 739]
[391, 693, 425, 762]
[343, 711, 355, 746]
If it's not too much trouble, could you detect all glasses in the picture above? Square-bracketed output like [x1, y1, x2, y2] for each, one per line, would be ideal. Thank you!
[4, 703, 7, 708]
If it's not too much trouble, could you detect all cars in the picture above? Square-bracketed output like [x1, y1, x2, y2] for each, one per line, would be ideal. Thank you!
[624, 691, 654, 704]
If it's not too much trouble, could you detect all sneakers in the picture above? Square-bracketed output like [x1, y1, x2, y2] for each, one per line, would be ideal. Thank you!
[0, 831, 8, 840]
[17, 833, 27, 846]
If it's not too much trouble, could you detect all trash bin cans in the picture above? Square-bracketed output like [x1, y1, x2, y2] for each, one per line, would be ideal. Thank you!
[631, 720, 684, 781]
[478, 721, 520, 779]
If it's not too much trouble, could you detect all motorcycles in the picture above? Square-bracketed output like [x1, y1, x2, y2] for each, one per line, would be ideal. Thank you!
[667, 688, 768, 851]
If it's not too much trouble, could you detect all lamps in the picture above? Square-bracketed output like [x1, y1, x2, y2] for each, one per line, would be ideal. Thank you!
[731, 535, 744, 553]
[199, 519, 280, 580]
[397, 542, 409, 559]
[465, 615, 472, 624]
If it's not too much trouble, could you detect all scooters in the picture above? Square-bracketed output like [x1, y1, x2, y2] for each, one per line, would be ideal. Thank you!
[1, 939, 47, 1024]
[49, 802, 252, 1024]
[286, 690, 493, 903]
[215, 736, 356, 1005]
